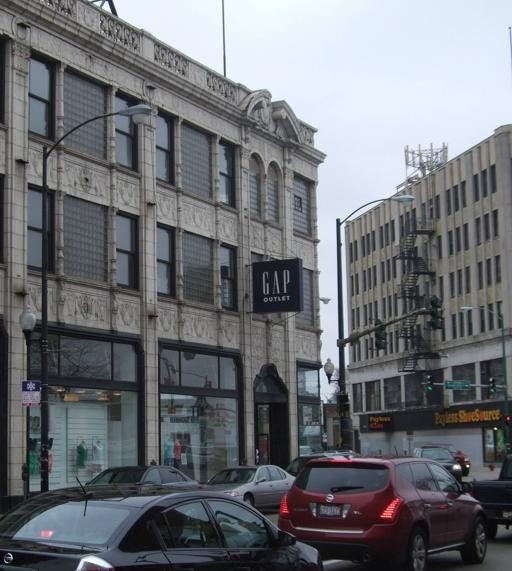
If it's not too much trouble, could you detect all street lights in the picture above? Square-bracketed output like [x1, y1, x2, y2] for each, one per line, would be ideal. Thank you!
[336, 195, 415, 393]
[460, 307, 509, 417]
[41, 104, 153, 494]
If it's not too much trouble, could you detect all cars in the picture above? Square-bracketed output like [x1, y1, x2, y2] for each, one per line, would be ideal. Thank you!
[86, 464, 201, 491]
[412, 443, 470, 484]
[1, 483, 325, 571]
[277, 457, 489, 571]
[203, 466, 297, 509]
[286, 451, 360, 475]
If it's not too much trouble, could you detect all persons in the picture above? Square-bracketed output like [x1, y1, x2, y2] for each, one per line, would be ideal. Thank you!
[164, 437, 182, 462]
[78, 439, 105, 472]
[29, 449, 53, 474]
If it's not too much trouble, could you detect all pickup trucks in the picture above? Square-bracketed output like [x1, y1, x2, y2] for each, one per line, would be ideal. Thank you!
[466, 453, 511, 538]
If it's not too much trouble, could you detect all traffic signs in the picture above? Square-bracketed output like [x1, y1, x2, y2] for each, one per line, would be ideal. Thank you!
[444, 381, 470, 390]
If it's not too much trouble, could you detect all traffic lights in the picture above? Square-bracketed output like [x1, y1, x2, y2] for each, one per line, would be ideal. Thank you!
[489, 377, 496, 395]
[425, 375, 432, 393]
